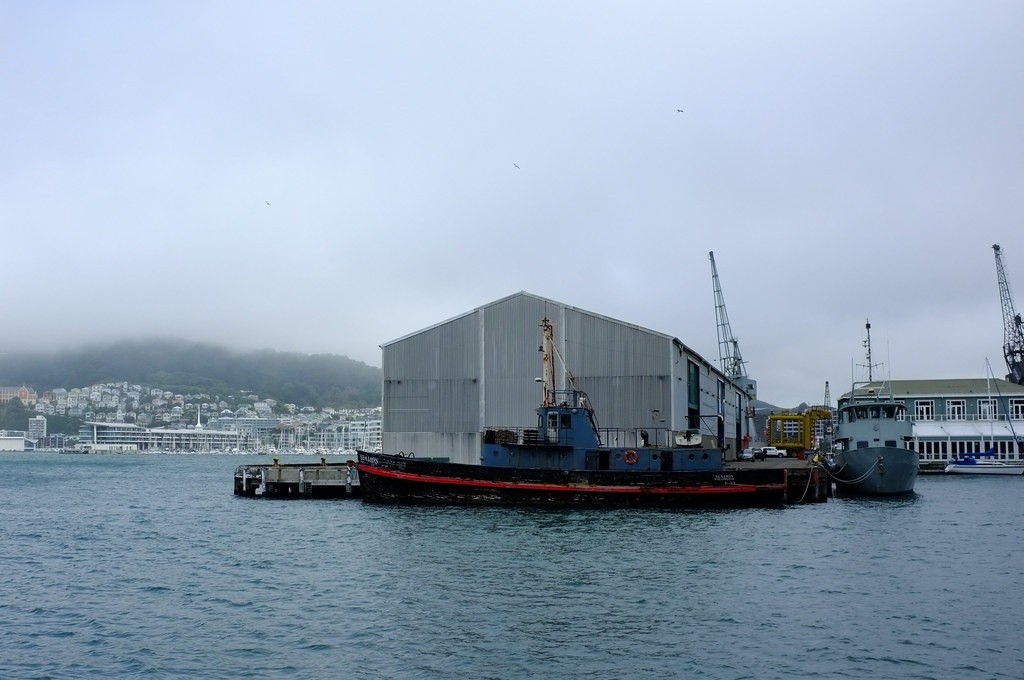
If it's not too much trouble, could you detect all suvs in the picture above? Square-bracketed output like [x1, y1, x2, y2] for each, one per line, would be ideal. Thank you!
[761, 447, 787, 458]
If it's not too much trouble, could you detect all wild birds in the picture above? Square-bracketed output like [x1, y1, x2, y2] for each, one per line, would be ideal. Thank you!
[675, 109, 685, 113]
[512, 162, 520, 170]
[264, 200, 271, 206]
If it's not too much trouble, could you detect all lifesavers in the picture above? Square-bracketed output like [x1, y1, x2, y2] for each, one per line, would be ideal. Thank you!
[624, 450, 639, 464]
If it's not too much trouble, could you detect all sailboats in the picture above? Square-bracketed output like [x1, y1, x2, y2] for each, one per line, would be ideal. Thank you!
[946, 357, 1024, 475]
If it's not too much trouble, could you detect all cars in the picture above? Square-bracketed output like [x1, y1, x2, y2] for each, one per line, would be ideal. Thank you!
[753, 449, 765, 461]
[739, 448, 754, 462]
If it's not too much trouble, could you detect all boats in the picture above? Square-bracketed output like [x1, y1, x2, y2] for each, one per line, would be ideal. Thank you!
[831, 319, 922, 496]
[354, 318, 787, 515]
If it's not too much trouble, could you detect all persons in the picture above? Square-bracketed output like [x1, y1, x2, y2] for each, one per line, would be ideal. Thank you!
[763, 448, 767, 461]
